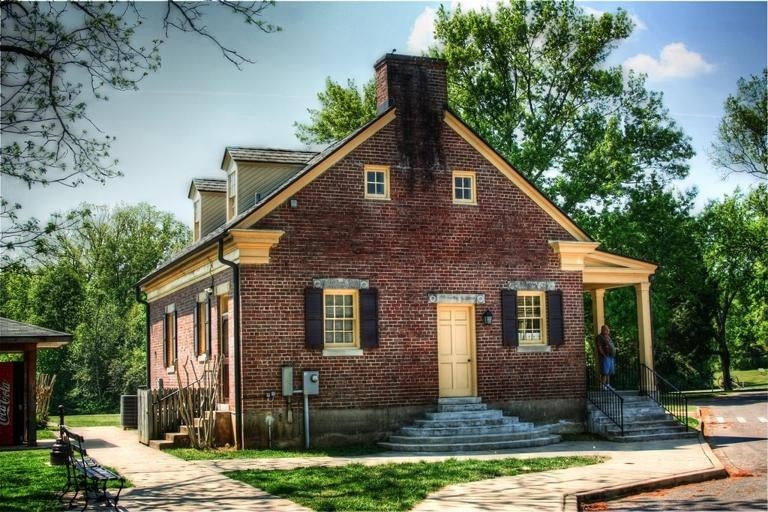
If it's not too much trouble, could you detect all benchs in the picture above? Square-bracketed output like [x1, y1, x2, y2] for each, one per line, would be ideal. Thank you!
[59, 426, 125, 512]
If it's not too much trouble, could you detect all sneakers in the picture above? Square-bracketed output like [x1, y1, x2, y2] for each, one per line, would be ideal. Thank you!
[600, 386, 615, 391]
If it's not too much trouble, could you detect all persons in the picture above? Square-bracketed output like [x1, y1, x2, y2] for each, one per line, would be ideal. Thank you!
[596, 325, 616, 391]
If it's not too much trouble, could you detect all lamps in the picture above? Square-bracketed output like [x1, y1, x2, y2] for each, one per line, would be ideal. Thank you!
[482, 310, 493, 325]
